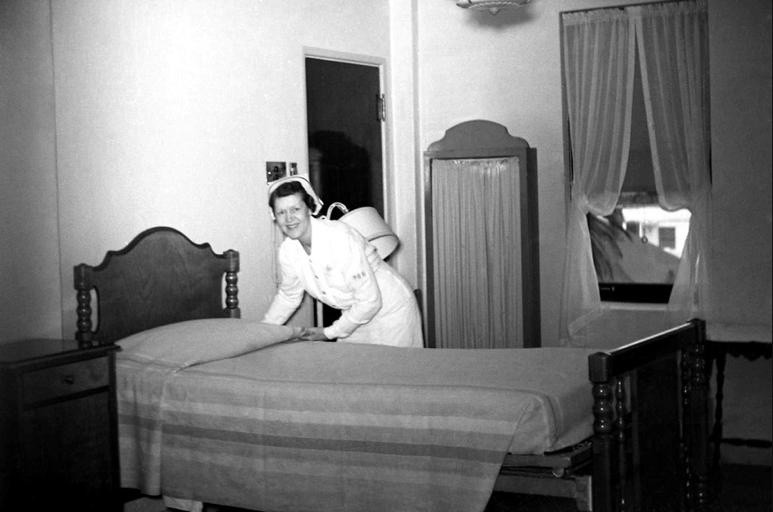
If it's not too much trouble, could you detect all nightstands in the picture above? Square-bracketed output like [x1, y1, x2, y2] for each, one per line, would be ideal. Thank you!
[1, 339, 124, 512]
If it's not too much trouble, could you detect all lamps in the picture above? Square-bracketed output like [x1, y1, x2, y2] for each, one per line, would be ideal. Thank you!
[316, 201, 402, 328]
[453, 0, 534, 15]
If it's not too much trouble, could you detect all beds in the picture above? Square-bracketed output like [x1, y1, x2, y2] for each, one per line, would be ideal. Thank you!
[72, 227, 710, 510]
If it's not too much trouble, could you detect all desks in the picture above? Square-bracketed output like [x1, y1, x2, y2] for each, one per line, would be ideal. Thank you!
[705, 320, 773, 451]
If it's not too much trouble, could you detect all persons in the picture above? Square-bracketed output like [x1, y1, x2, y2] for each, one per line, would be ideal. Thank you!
[261, 177, 424, 348]
